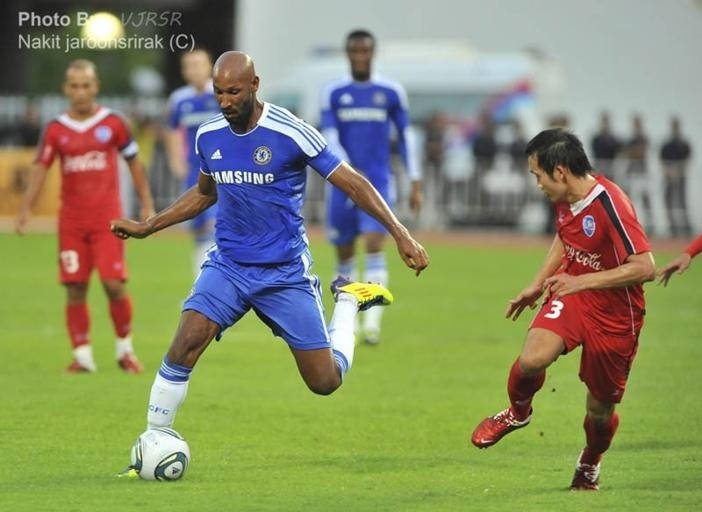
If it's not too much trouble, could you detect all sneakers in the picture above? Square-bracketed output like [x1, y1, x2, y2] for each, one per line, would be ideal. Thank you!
[116, 467, 140, 479]
[117, 353, 142, 372]
[67, 358, 89, 372]
[330, 275, 394, 311]
[570, 447, 603, 492]
[472, 406, 533, 448]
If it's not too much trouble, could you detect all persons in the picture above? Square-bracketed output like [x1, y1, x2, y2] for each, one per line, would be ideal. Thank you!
[434, 80, 588, 237]
[588, 111, 619, 181]
[657, 116, 693, 236]
[13, 56, 157, 375]
[163, 49, 223, 275]
[657, 233, 702, 288]
[316, 28, 425, 349]
[108, 48, 432, 477]
[468, 126, 657, 491]
[620, 110, 654, 233]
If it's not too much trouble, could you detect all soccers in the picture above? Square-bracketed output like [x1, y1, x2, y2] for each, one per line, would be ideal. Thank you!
[130, 426, 191, 481]
